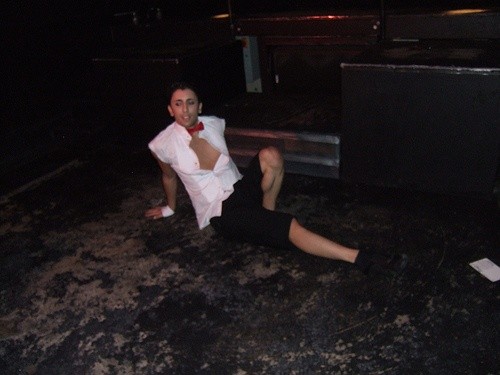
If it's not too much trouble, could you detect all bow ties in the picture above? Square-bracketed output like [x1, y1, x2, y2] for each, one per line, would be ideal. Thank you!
[187, 122, 204, 135]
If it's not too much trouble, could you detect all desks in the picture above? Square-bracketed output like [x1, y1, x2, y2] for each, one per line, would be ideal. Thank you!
[338, 37, 499, 203]
[81, 38, 244, 164]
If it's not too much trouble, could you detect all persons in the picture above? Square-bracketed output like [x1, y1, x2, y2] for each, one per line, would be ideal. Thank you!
[144, 81, 379, 278]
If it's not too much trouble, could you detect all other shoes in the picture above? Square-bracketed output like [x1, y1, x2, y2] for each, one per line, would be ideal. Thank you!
[358, 252, 409, 278]
